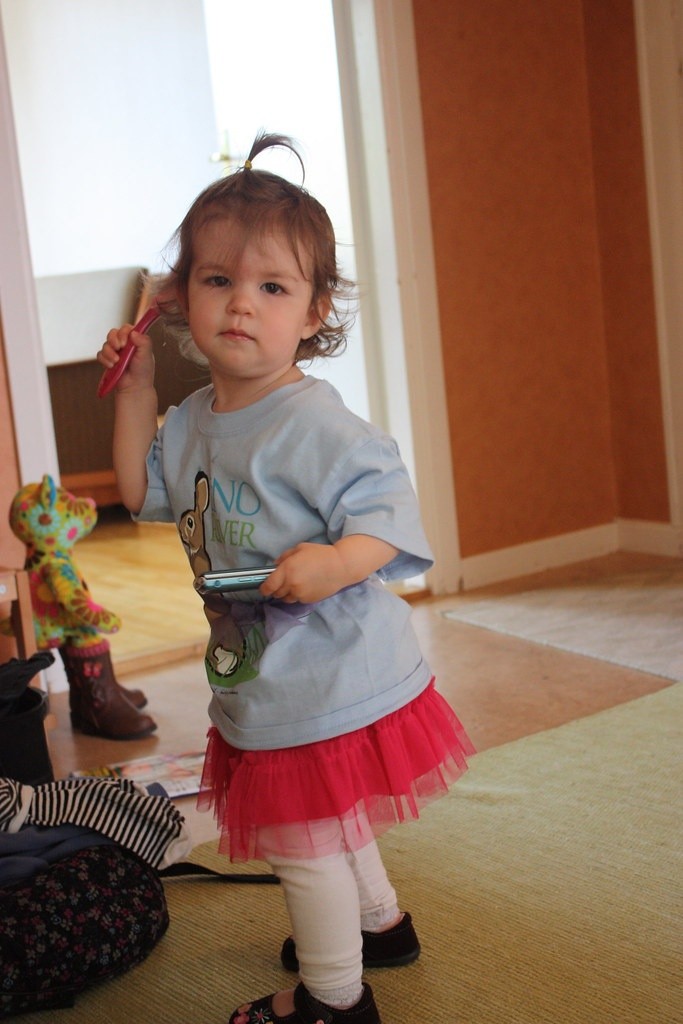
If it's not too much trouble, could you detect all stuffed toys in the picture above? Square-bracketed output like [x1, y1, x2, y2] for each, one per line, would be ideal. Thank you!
[7, 474, 156, 738]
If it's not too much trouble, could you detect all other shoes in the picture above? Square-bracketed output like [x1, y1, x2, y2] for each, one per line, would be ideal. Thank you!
[229, 980, 382, 1024]
[280, 912, 421, 972]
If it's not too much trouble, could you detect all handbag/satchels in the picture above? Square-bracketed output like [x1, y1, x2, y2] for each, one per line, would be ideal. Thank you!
[0, 826, 170, 1016]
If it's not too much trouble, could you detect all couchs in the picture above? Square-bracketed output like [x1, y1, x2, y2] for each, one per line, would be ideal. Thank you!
[35, 265, 170, 513]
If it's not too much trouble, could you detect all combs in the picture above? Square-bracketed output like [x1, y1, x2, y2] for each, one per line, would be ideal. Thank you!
[98, 291, 174, 398]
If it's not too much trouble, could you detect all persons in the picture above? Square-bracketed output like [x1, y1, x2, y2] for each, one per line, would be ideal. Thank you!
[96, 134, 477, 1024]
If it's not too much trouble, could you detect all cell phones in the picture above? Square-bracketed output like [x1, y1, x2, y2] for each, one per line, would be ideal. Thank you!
[193, 565, 278, 595]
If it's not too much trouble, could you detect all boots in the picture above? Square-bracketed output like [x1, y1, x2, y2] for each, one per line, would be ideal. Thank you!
[58, 638, 157, 740]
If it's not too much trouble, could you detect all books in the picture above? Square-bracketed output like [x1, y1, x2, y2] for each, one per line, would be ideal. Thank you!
[69, 748, 216, 805]
